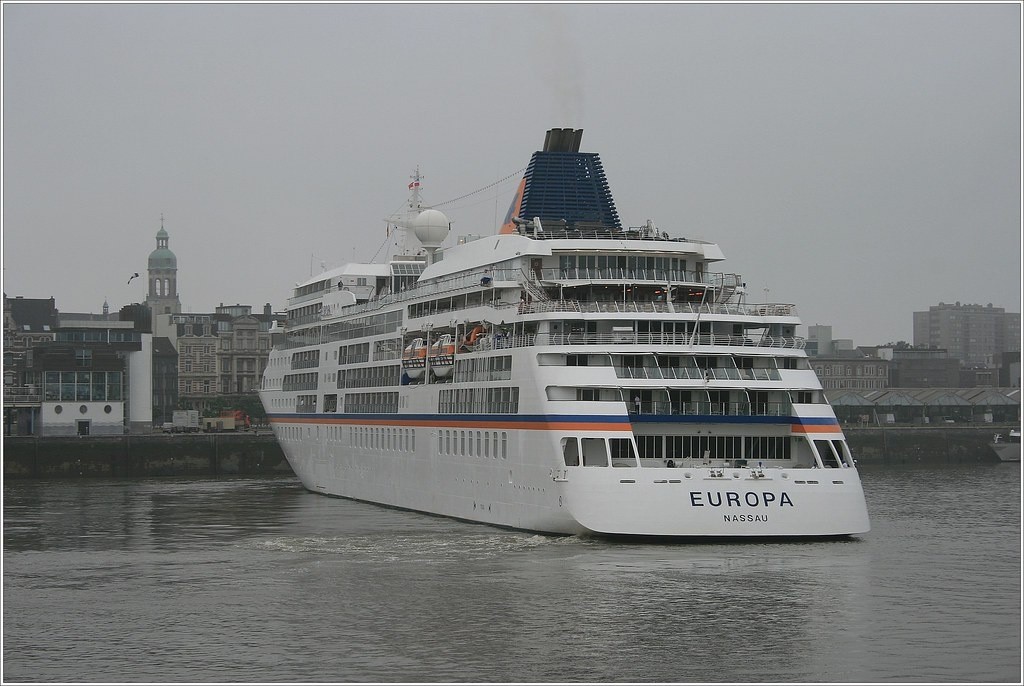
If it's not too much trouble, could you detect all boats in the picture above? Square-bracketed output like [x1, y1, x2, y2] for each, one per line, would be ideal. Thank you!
[402, 336, 436, 380]
[428, 334, 464, 378]
[462, 325, 494, 351]
[987, 430, 1021, 462]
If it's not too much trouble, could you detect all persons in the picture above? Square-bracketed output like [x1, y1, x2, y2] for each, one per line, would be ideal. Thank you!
[634, 395, 639, 415]
[841, 455, 857, 468]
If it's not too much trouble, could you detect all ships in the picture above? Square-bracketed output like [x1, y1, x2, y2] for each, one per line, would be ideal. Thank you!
[252, 126, 872, 540]
